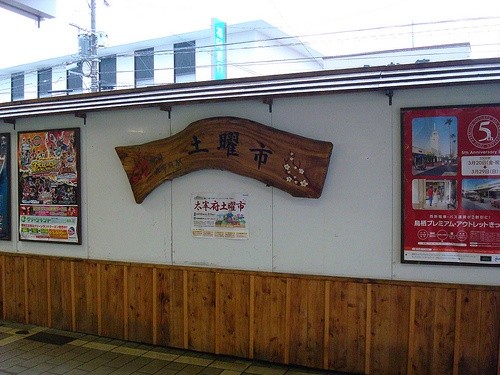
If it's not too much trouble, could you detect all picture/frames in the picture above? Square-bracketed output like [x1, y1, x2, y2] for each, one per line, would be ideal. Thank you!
[0, 131, 12, 241]
[16, 127, 82, 246]
[400, 103, 500, 268]
